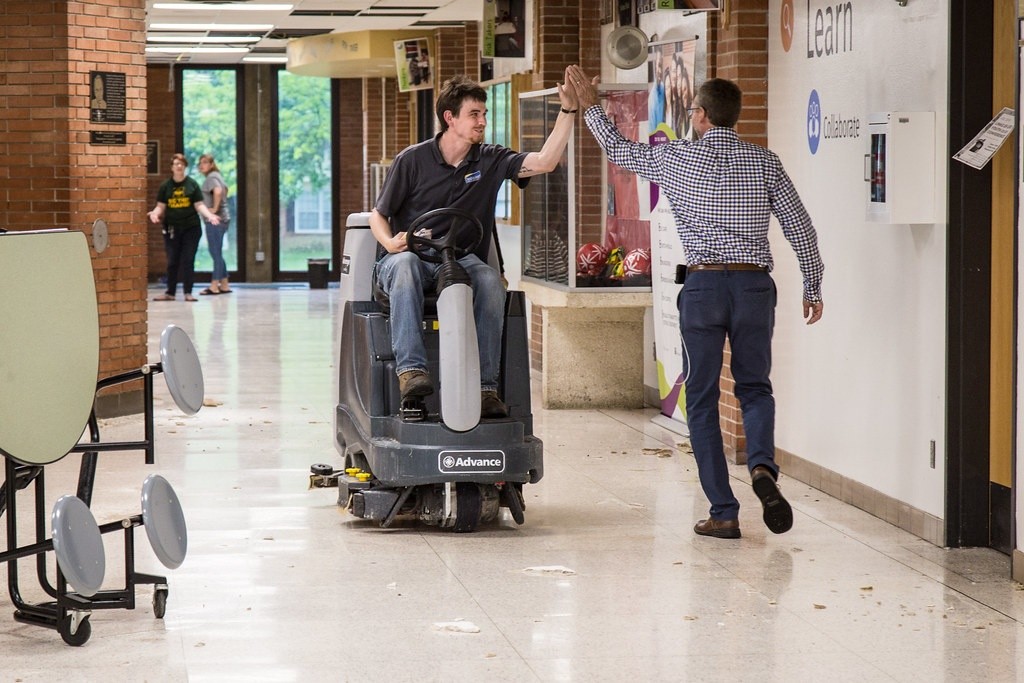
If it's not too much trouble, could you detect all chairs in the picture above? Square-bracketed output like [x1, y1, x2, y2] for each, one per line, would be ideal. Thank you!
[384, 279, 508, 420]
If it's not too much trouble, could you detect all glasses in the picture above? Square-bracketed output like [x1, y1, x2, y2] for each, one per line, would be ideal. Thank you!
[686, 107, 700, 119]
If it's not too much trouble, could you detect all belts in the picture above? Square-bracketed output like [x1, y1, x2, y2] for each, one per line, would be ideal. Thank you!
[687, 263, 771, 272]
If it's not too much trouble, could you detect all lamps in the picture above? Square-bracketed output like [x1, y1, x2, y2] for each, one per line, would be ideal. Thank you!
[150, 23, 275, 29]
[145, 36, 261, 44]
[151, 3, 294, 11]
[241, 56, 288, 64]
[145, 47, 250, 53]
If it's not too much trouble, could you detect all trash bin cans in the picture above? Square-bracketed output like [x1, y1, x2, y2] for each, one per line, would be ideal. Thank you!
[336, 212, 503, 440]
[308, 258, 330, 289]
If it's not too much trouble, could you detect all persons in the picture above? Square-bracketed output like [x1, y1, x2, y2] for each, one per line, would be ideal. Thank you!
[196, 153, 232, 296]
[370, 65, 581, 420]
[567, 64, 827, 539]
[146, 153, 223, 303]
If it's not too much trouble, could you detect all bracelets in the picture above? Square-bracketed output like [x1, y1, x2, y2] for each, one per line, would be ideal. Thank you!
[560, 106, 578, 115]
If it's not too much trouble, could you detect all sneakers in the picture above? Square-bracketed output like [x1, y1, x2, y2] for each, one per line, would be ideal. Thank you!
[397, 369, 436, 402]
[481, 390, 507, 418]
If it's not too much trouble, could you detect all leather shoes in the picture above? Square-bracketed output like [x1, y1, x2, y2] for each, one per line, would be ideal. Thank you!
[694, 516, 743, 538]
[751, 467, 795, 534]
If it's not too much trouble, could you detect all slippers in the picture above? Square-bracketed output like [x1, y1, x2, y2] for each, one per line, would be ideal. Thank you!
[185, 296, 198, 302]
[152, 293, 176, 301]
[200, 284, 232, 295]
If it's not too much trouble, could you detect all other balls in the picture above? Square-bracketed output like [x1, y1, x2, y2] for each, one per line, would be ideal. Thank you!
[623, 247, 651, 278]
[577, 241, 608, 274]
